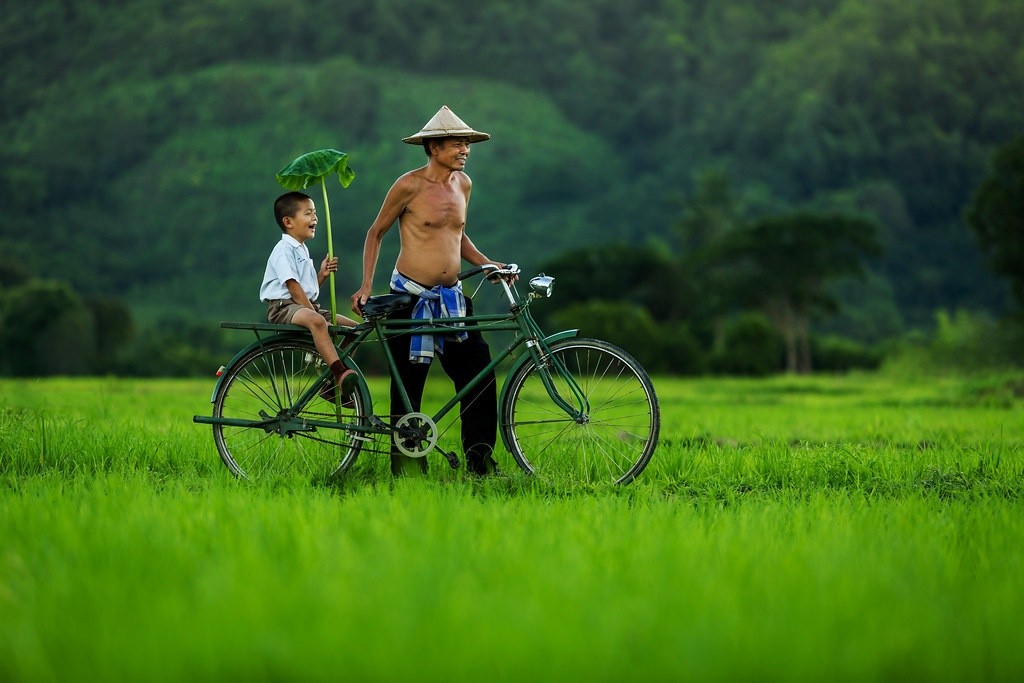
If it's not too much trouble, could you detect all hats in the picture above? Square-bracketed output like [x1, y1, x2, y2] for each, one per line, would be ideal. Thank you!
[402, 106, 490, 146]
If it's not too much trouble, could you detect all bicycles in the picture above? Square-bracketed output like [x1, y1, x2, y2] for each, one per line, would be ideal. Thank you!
[193, 264, 660, 488]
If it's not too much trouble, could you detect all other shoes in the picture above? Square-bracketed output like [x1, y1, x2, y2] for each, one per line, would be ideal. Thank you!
[331, 359, 359, 394]
[318, 384, 356, 408]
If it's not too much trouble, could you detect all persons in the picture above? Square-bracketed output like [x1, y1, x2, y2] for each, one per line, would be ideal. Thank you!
[350, 106, 519, 479]
[259, 191, 361, 409]
